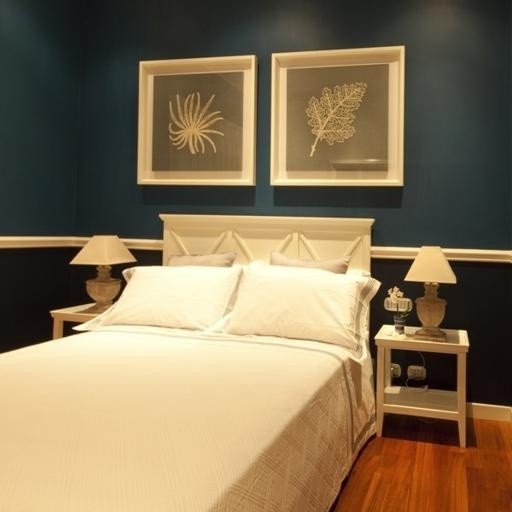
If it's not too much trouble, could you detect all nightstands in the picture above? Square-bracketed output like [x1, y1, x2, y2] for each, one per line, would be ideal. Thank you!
[47, 302, 106, 339]
[372, 325, 471, 451]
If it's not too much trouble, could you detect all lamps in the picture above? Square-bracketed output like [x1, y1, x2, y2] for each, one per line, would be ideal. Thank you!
[68, 233, 140, 311]
[403, 243, 458, 342]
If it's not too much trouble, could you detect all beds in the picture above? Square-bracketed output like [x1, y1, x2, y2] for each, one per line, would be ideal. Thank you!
[0, 210, 378, 512]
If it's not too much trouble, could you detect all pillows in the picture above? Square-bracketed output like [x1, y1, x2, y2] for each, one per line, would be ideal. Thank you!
[106, 263, 381, 363]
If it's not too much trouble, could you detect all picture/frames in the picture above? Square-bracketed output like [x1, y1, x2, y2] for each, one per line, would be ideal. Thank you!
[135, 55, 257, 188]
[268, 45, 406, 189]
